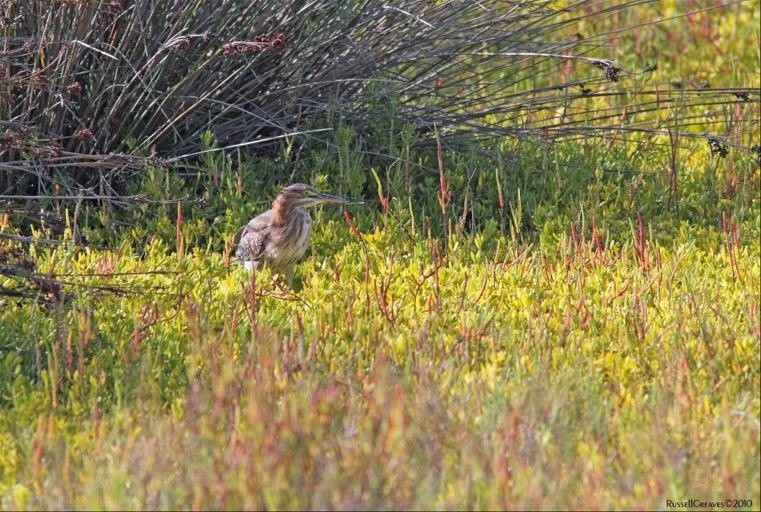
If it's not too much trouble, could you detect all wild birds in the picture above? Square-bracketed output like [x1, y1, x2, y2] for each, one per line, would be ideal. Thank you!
[237, 183, 370, 291]
